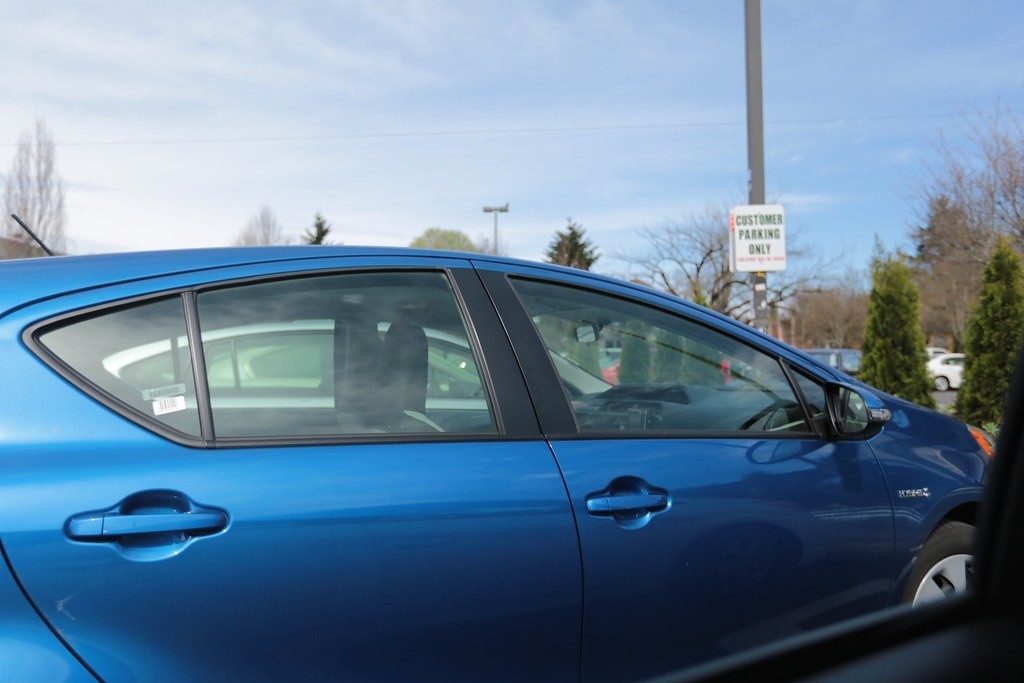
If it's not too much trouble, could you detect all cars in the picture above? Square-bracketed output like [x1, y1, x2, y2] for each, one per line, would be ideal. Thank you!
[0, 247, 999, 683]
[925, 353, 966, 391]
